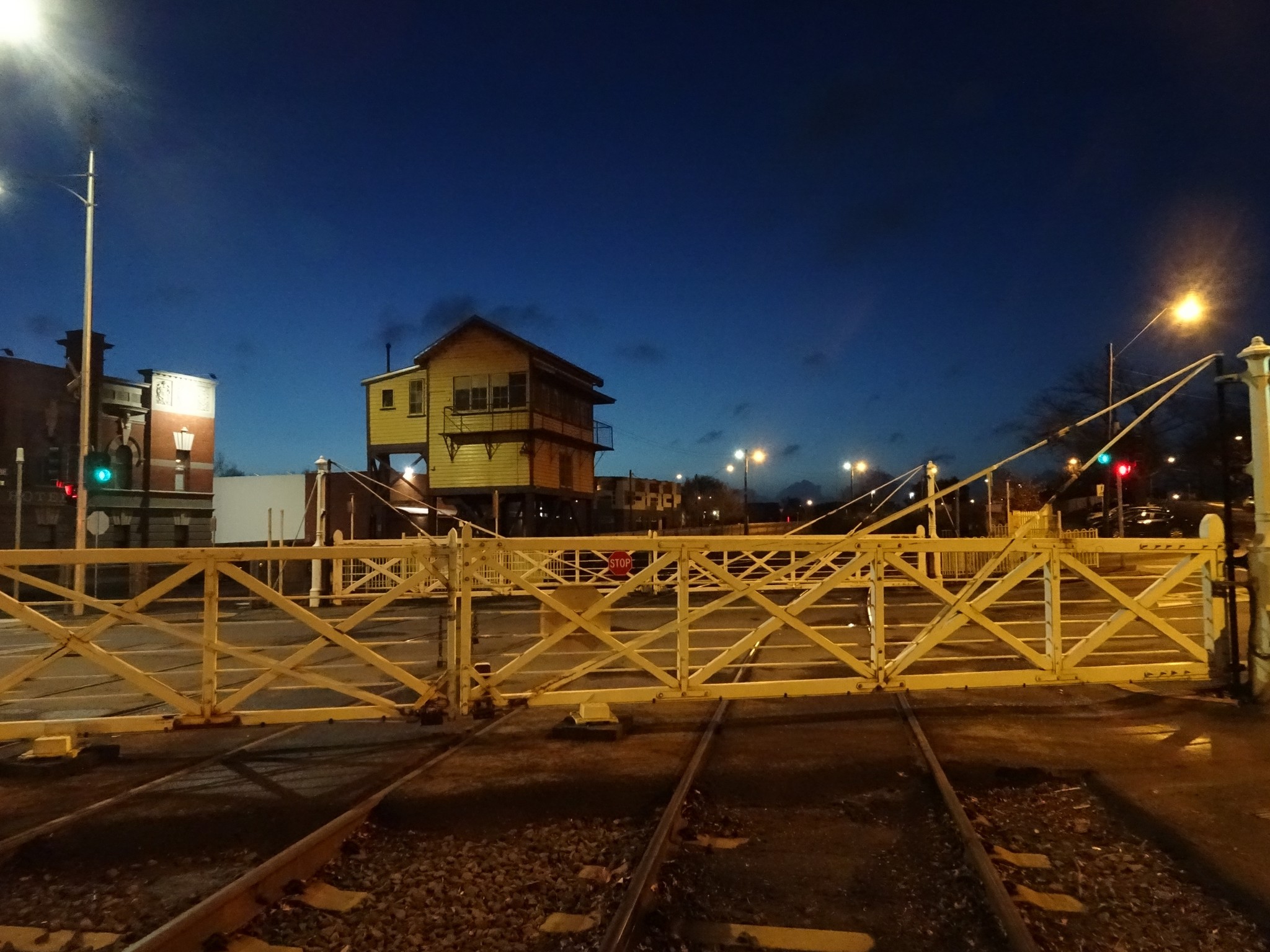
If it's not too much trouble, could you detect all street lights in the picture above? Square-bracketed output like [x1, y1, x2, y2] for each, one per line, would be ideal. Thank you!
[1102, 286, 1208, 550]
[734, 448, 767, 535]
[843, 462, 866, 502]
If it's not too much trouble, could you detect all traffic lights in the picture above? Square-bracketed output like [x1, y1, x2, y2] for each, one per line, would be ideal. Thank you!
[1097, 452, 1111, 469]
[86, 450, 112, 487]
[1115, 458, 1130, 480]
[47, 444, 62, 485]
[62, 484, 77, 499]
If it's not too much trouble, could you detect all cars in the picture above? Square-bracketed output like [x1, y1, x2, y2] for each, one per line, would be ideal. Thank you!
[1243, 495, 1254, 511]
[1082, 501, 1194, 538]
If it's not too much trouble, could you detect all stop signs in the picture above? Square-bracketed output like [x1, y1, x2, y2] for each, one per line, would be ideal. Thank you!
[608, 551, 633, 576]
